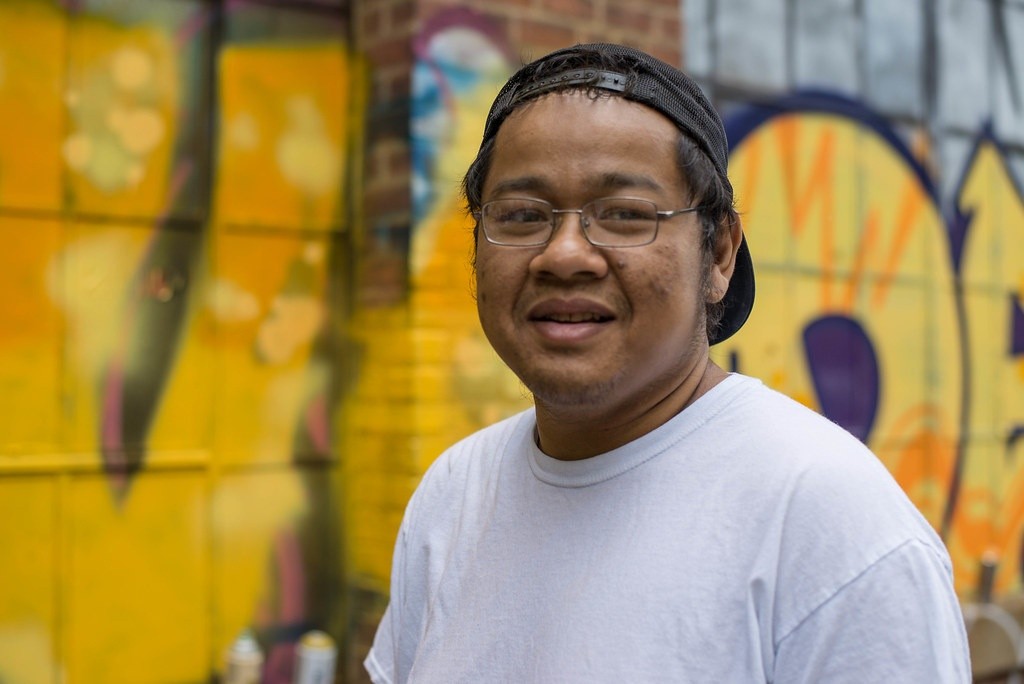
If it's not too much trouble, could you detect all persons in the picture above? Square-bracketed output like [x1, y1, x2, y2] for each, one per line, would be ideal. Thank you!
[364, 44, 972, 683]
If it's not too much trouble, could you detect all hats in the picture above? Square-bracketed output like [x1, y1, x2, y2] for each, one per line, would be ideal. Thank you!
[476, 43, 756, 347]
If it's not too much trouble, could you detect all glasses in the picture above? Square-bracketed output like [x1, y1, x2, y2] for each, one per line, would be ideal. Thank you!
[472, 197, 710, 248]
